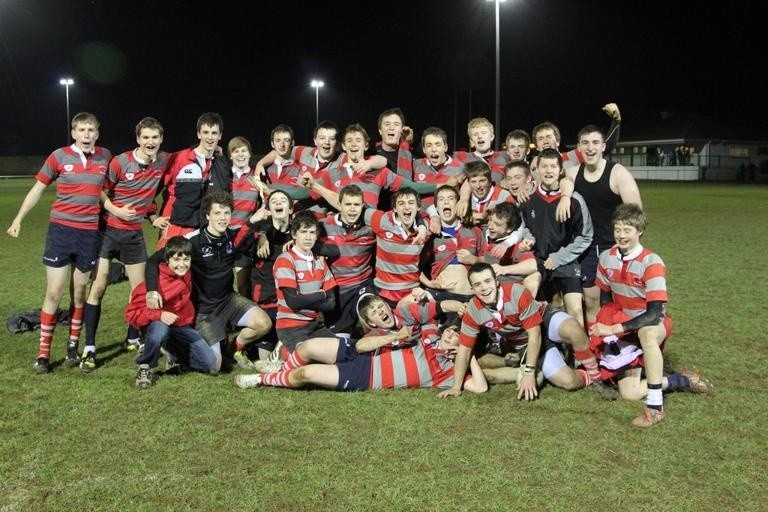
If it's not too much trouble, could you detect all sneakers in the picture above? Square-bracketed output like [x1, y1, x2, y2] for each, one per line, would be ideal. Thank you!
[124, 338, 140, 350]
[227, 340, 284, 390]
[135, 364, 152, 389]
[681, 369, 713, 393]
[632, 407, 664, 428]
[34, 357, 49, 373]
[79, 351, 96, 373]
[66, 351, 81, 364]
[594, 381, 618, 401]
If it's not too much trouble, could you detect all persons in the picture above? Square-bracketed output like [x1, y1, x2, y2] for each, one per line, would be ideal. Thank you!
[5, 102, 708, 428]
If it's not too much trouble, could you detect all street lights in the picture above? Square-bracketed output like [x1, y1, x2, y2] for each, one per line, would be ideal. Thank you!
[486, 0, 506, 150]
[309, 79, 326, 126]
[59, 78, 75, 146]
